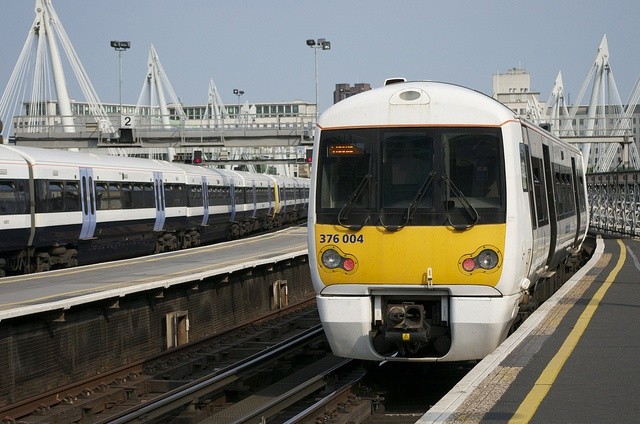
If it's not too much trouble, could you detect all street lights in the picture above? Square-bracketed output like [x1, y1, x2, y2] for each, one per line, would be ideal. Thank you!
[233, 87, 245, 113]
[306, 36, 330, 118]
[110, 39, 131, 113]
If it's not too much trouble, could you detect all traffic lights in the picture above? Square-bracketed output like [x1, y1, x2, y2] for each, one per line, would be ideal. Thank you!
[539, 122, 550, 132]
[306, 148, 313, 162]
[193, 151, 202, 163]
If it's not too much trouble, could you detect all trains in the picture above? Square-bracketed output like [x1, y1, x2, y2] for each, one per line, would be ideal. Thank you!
[0, 144, 309, 274]
[305, 80, 590, 361]
